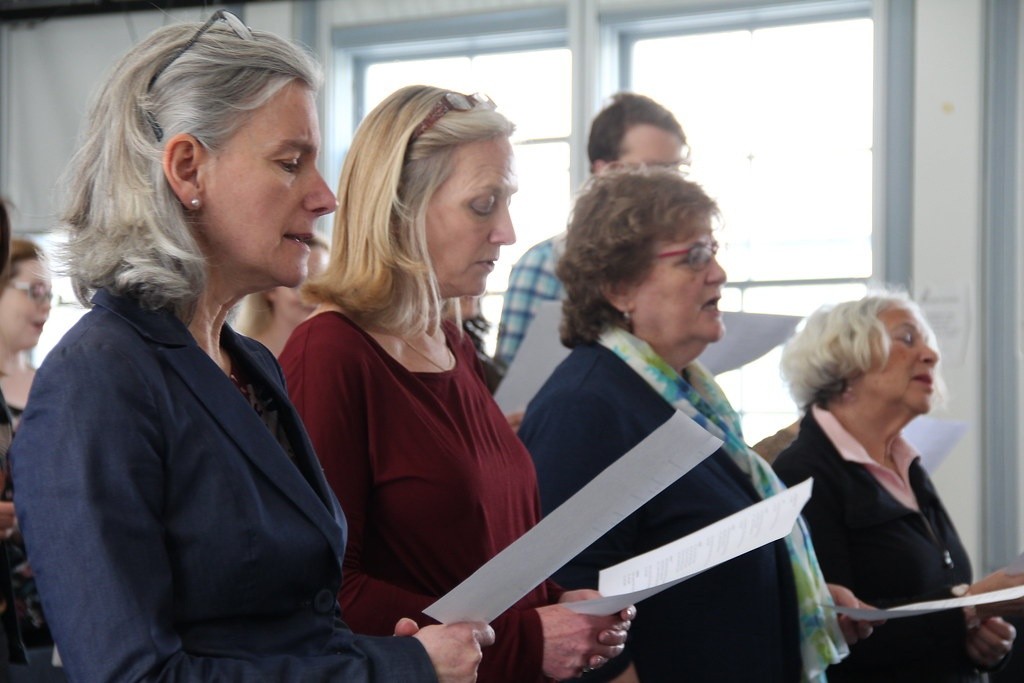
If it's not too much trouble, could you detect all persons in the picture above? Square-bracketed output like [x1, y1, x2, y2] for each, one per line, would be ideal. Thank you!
[445, 293, 523, 436]
[7, 24, 495, 683]
[277, 84, 636, 683]
[518, 164, 885, 683]
[0, 197, 56, 683]
[241, 236, 331, 361]
[772, 294, 1024, 683]
[496, 88, 799, 461]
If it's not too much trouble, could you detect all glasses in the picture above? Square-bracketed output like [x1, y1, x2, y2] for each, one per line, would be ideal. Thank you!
[408, 92, 497, 148]
[145, 9, 253, 141]
[652, 242, 718, 269]
[8, 281, 53, 303]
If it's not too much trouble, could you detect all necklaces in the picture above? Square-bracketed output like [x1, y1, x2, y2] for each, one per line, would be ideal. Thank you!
[392, 330, 453, 371]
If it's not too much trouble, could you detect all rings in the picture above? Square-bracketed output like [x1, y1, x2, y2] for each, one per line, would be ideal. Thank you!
[581, 667, 590, 673]
